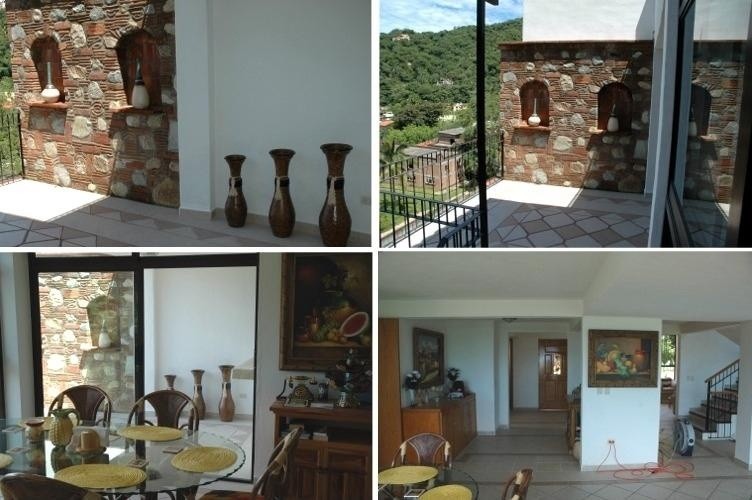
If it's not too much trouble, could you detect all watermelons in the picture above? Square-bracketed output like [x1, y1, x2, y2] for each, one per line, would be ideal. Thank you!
[341, 311, 371, 340]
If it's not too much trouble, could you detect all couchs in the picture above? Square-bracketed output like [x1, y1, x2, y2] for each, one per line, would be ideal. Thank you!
[661, 378, 677, 404]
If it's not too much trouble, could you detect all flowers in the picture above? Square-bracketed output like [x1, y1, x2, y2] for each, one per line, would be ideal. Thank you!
[402, 371, 423, 391]
[446, 368, 460, 382]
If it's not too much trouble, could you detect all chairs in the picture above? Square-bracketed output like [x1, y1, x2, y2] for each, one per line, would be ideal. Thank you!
[0, 472, 107, 500]
[389, 433, 453, 495]
[125, 390, 199, 500]
[198, 427, 303, 500]
[501, 468, 533, 500]
[48, 385, 112, 447]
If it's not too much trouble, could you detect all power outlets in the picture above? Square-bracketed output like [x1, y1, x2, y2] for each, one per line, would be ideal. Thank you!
[608, 437, 615, 445]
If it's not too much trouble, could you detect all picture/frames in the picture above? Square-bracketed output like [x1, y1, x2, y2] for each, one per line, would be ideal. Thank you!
[588, 329, 659, 387]
[413, 327, 444, 390]
[279, 253, 372, 372]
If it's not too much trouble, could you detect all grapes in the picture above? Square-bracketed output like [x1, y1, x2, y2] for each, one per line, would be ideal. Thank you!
[315, 318, 340, 342]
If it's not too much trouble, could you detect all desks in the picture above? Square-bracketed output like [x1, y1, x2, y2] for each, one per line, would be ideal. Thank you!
[0, 425, 246, 500]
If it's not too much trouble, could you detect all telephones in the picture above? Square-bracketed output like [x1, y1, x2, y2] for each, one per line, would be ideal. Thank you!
[276, 376, 318, 402]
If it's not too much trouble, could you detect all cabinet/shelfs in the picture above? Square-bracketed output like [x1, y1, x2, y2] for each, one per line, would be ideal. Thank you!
[270, 406, 372, 500]
[402, 393, 476, 464]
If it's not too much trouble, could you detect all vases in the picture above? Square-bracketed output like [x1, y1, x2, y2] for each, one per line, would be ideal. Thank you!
[319, 143, 353, 247]
[164, 375, 176, 390]
[447, 380, 455, 391]
[269, 149, 296, 238]
[191, 370, 206, 420]
[218, 365, 235, 422]
[224, 154, 247, 228]
[408, 389, 416, 405]
